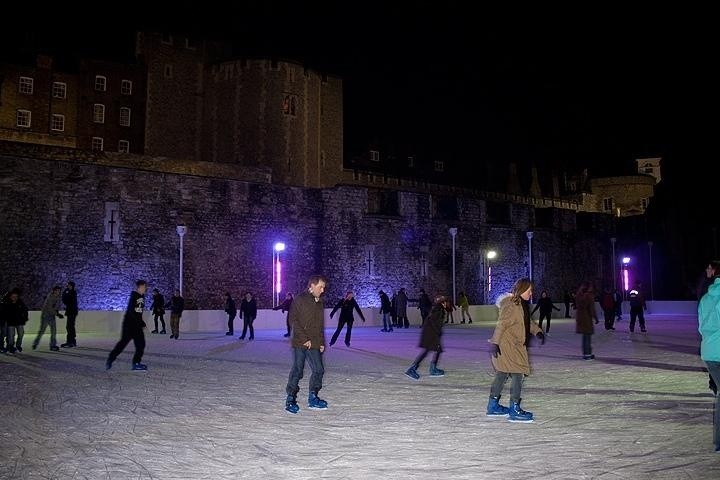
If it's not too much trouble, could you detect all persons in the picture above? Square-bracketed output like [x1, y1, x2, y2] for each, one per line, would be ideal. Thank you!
[273, 293, 294, 337]
[285, 274, 327, 411]
[165, 289, 185, 340]
[697, 260, 718, 395]
[697, 262, 720, 449]
[105, 280, 148, 369]
[0, 281, 78, 353]
[329, 291, 365, 346]
[488, 277, 544, 418]
[378, 287, 472, 332]
[149, 287, 166, 334]
[530, 279, 647, 360]
[239, 291, 257, 340]
[224, 292, 237, 336]
[407, 298, 447, 378]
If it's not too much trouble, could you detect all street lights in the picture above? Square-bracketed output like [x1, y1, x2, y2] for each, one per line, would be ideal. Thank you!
[483, 249, 496, 305]
[273, 243, 285, 309]
[177, 226, 187, 297]
[527, 231, 534, 303]
[450, 228, 458, 307]
[620, 257, 631, 301]
[648, 242, 655, 301]
[611, 238, 618, 300]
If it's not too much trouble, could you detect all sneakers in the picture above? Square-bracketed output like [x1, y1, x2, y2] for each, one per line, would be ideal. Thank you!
[131, 360, 148, 370]
[583, 354, 595, 360]
[105, 358, 113, 370]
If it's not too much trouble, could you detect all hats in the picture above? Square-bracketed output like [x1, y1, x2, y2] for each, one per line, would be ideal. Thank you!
[434, 295, 448, 304]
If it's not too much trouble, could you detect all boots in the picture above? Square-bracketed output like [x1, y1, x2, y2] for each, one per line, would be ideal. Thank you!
[284, 394, 301, 413]
[307, 390, 329, 408]
[428, 361, 445, 375]
[509, 398, 535, 420]
[403, 361, 421, 380]
[486, 395, 510, 414]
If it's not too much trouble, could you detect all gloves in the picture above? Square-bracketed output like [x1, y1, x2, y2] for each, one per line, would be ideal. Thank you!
[535, 330, 546, 346]
[487, 342, 502, 360]
